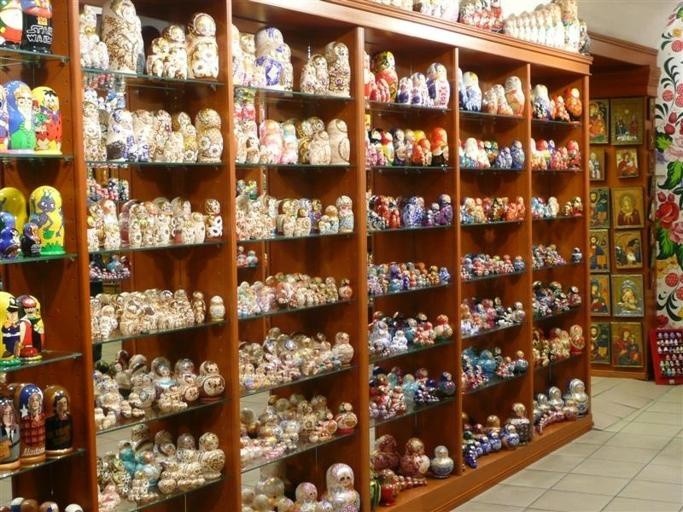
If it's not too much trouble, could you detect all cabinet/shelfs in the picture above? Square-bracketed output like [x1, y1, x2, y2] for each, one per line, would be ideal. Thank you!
[528, 58, 591, 440]
[457, 42, 532, 477]
[358, 16, 462, 508]
[76, 0, 240, 511]
[583, 93, 661, 377]
[228, 0, 367, 512]
[1, 0, 95, 512]
